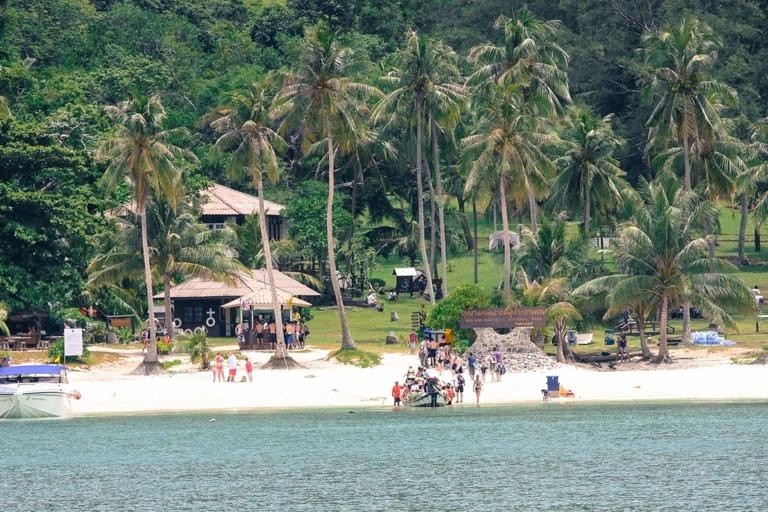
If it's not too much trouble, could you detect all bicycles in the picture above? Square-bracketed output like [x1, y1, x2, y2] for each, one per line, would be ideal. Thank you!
[199, 359, 210, 370]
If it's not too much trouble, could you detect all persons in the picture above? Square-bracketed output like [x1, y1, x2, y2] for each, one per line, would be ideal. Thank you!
[623, 307, 630, 323]
[392, 329, 506, 407]
[618, 334, 632, 362]
[234, 318, 308, 350]
[141, 327, 171, 354]
[211, 352, 253, 383]
[367, 287, 402, 312]
[750, 285, 761, 306]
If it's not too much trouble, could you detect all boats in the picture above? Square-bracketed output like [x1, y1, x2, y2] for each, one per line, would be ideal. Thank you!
[0, 366, 80, 419]
[399, 387, 448, 406]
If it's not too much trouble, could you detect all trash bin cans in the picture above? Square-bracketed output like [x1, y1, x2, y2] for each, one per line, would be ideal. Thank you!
[605, 329, 614, 344]
